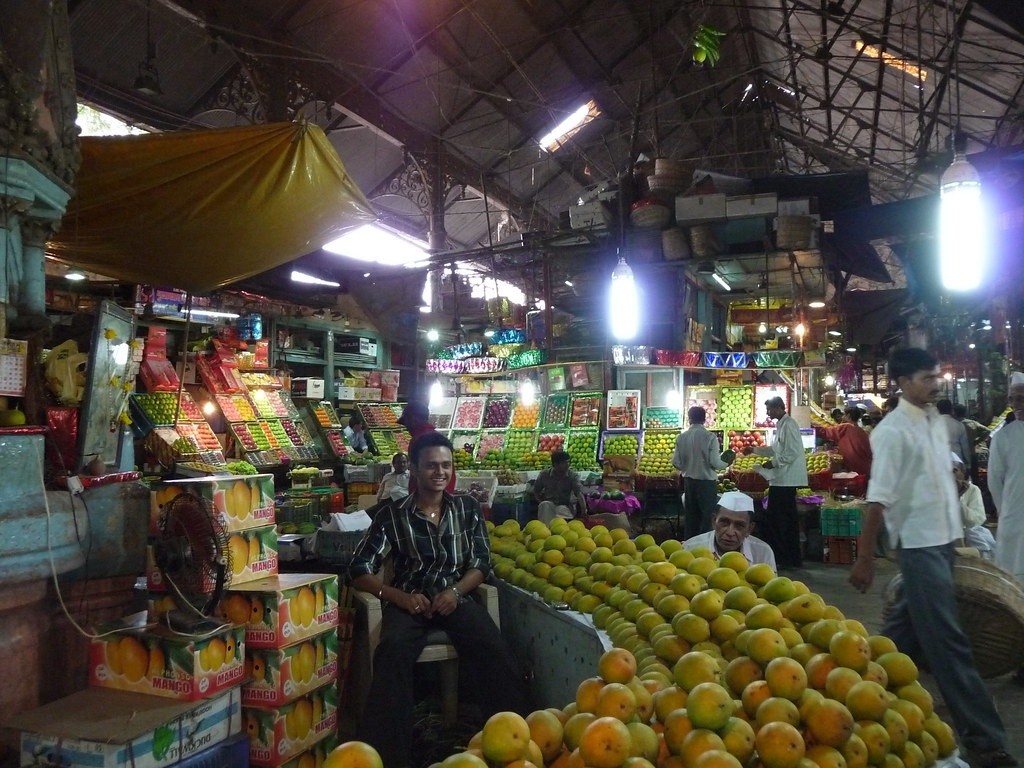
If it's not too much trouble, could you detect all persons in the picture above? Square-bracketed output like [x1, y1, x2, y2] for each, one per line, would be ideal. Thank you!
[534, 452, 589, 526]
[987, 371, 1024, 574]
[850, 348, 1022, 768]
[938, 398, 994, 564]
[682, 492, 778, 577]
[672, 407, 733, 542]
[740, 397, 808, 569]
[396, 402, 456, 494]
[348, 434, 526, 768]
[344, 417, 368, 452]
[366, 454, 410, 518]
[812, 396, 899, 558]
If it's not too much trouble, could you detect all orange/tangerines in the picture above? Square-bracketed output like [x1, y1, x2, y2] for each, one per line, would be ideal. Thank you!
[103, 481, 326, 768]
[314, 408, 331, 427]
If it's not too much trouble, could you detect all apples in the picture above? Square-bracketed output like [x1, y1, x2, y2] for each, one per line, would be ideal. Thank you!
[133, 393, 221, 455]
[213, 393, 303, 450]
[357, 405, 413, 456]
[690, 387, 829, 494]
[330, 432, 348, 457]
[988, 407, 1012, 430]
[452, 398, 676, 476]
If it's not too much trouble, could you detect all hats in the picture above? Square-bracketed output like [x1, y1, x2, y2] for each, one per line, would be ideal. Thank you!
[717, 491, 755, 513]
[1011, 372, 1024, 385]
[952, 451, 965, 464]
[395, 401, 429, 424]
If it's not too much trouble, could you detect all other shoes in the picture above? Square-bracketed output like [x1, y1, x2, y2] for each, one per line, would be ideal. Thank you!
[966, 748, 1019, 768]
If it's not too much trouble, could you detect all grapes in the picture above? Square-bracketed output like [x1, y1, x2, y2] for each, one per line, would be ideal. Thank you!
[225, 460, 260, 475]
[795, 488, 815, 496]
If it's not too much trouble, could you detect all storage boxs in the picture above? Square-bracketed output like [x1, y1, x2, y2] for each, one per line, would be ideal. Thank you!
[348, 578, 501, 724]
[239, 675, 341, 767]
[89, 616, 247, 701]
[7, 684, 242, 768]
[729, 471, 768, 492]
[777, 198, 810, 218]
[773, 213, 821, 251]
[237, 626, 341, 704]
[278, 729, 341, 768]
[167, 733, 250, 768]
[569, 204, 611, 230]
[726, 193, 778, 217]
[803, 350, 826, 366]
[674, 194, 726, 221]
[809, 472, 831, 490]
[213, 572, 340, 647]
[827, 539, 854, 563]
[821, 508, 861, 537]
[163, 473, 276, 533]
[432, 384, 839, 495]
[832, 474, 865, 500]
[202, 524, 280, 592]
[127, 355, 414, 478]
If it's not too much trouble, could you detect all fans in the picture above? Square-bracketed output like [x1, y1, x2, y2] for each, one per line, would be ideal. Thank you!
[153, 493, 234, 636]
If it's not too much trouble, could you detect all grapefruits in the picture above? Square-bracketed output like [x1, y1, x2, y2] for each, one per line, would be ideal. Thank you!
[0, 408, 26, 426]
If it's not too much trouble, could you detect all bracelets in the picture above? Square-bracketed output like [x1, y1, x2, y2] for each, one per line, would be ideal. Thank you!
[448, 587, 462, 604]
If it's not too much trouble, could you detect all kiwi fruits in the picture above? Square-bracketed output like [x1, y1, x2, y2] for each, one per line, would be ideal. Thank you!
[495, 470, 520, 485]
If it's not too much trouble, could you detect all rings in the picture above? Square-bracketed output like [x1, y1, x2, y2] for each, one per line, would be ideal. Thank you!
[415, 605, 419, 609]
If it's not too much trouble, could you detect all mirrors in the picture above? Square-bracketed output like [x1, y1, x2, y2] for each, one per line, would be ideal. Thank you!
[73, 301, 140, 474]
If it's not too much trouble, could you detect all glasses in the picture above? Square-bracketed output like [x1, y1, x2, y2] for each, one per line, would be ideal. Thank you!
[952, 468, 961, 474]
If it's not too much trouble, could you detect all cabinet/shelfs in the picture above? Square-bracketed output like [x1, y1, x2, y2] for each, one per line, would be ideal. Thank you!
[263, 315, 388, 403]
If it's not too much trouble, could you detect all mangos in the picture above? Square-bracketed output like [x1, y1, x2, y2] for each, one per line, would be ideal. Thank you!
[326, 515, 957, 768]
[591, 489, 626, 499]
